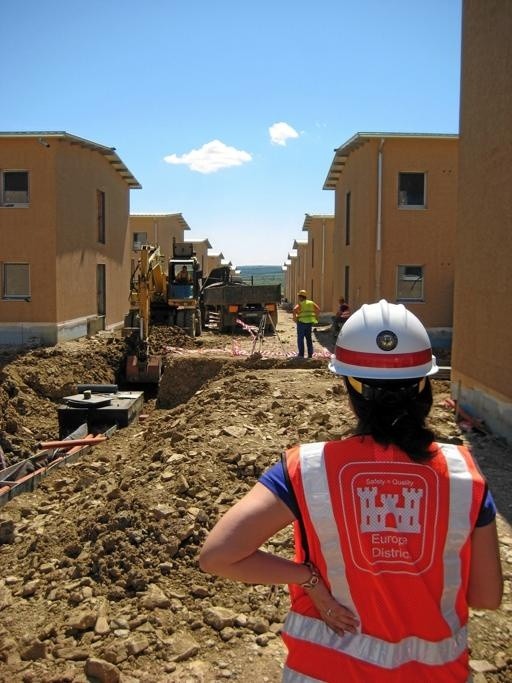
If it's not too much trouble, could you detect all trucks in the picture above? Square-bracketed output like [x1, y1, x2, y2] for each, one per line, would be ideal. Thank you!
[196, 264, 283, 335]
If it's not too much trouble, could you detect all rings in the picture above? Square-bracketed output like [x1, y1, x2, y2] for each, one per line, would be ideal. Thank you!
[327, 609, 331, 616]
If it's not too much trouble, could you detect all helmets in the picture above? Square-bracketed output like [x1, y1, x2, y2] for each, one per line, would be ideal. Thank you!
[297, 289, 307, 296]
[328, 299, 440, 379]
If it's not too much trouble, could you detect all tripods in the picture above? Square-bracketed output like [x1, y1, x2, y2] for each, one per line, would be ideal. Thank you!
[252, 314, 288, 360]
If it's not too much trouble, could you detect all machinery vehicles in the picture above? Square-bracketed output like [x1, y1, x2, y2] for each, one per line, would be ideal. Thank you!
[118, 241, 211, 385]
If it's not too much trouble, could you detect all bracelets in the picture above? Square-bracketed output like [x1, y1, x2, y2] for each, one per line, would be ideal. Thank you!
[302, 561, 321, 591]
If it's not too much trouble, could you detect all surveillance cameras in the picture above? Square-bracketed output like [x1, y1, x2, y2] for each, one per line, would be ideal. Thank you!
[38, 140, 50, 148]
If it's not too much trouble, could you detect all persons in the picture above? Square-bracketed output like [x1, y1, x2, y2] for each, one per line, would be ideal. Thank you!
[175, 266, 192, 283]
[294, 290, 321, 358]
[198, 300, 504, 683]
[331, 297, 351, 337]
